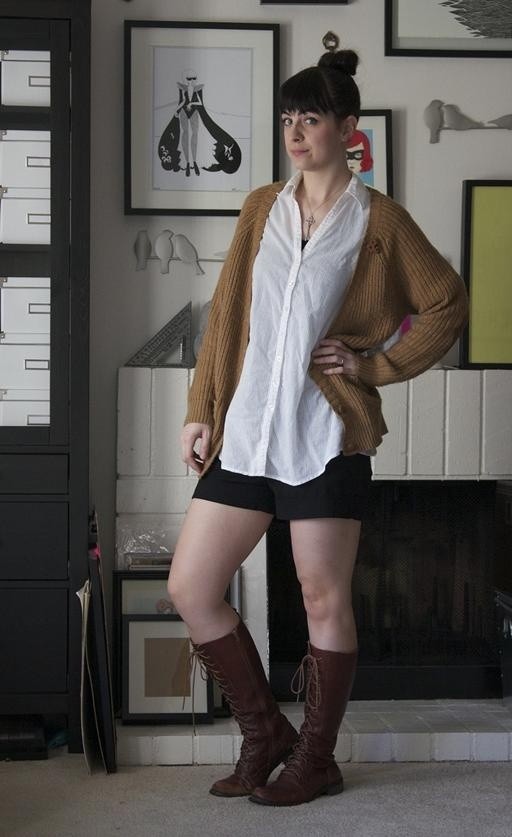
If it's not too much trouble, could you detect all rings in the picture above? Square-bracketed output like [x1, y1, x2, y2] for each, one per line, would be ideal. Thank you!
[342, 359, 344, 364]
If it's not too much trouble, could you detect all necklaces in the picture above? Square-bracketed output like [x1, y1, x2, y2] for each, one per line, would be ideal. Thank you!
[302, 173, 356, 241]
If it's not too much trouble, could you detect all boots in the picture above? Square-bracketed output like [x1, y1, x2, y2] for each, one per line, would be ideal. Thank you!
[181, 607, 292, 807]
[249, 641, 360, 805]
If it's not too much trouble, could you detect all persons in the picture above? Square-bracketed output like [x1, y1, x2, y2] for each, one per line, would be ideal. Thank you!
[345, 130, 373, 174]
[167, 50, 469, 806]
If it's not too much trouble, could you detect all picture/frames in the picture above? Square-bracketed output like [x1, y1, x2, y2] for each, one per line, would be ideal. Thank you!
[110, 545, 244, 726]
[383, 0, 512, 57]
[457, 179, 511, 370]
[344, 108, 393, 200]
[123, 18, 281, 217]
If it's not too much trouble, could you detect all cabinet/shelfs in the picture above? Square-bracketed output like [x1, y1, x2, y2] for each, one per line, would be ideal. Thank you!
[0, 0, 90, 758]
